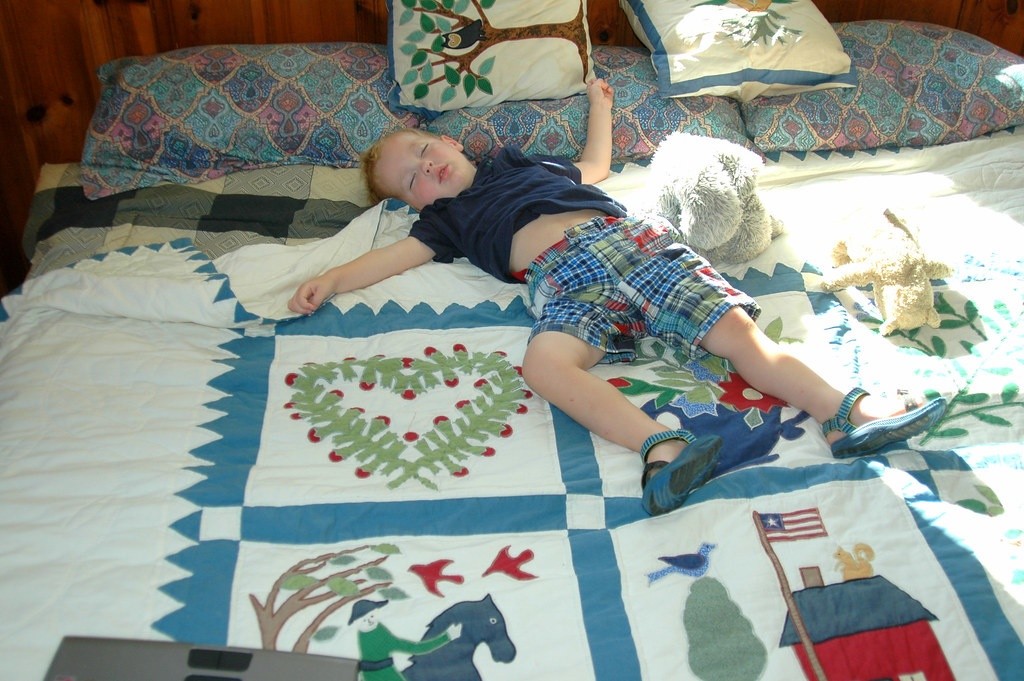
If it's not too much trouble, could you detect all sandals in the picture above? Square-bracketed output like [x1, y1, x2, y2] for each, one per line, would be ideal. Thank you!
[641, 429, 723, 517]
[822, 388, 945, 459]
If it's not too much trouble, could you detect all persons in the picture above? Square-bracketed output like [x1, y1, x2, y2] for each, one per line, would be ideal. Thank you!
[289, 78, 948, 519]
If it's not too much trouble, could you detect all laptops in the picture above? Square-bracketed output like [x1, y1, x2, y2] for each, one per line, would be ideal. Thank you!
[43, 637, 360, 681]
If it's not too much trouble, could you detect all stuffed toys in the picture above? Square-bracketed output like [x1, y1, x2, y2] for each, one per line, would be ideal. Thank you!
[820, 210, 950, 335]
[637, 128, 783, 265]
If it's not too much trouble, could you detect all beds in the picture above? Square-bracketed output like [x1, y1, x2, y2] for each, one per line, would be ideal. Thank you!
[0, 164, 1024, 681]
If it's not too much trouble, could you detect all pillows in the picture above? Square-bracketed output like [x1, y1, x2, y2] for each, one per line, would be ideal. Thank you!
[425, 50, 754, 169]
[739, 20, 1024, 148]
[381, 0, 597, 112]
[82, 42, 417, 203]
[622, 0, 860, 102]
